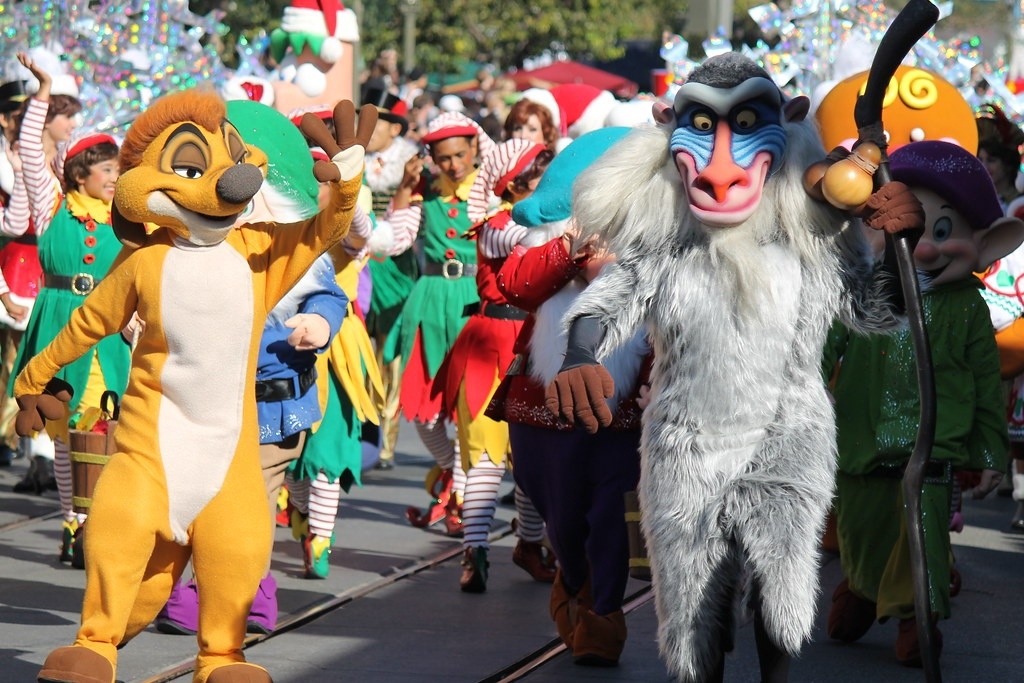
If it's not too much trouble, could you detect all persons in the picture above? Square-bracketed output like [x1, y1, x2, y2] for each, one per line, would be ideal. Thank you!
[973, 109, 1024, 219]
[364, 44, 403, 110]
[0, 54, 564, 595]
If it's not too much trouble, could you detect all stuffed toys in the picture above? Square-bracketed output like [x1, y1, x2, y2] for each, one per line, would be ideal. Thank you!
[13, 88, 379, 683]
[497, 51, 1024, 683]
[266, 0, 358, 115]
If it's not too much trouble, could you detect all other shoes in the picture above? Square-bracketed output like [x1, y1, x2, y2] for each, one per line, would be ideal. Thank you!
[275, 483, 336, 580]
[58, 518, 86, 571]
[458, 543, 492, 593]
[12, 455, 58, 497]
[405, 464, 464, 539]
[511, 532, 559, 584]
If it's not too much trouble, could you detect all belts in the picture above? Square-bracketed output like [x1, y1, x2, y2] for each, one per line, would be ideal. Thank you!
[10, 234, 38, 246]
[460, 300, 529, 322]
[255, 365, 319, 403]
[43, 273, 102, 295]
[420, 259, 478, 280]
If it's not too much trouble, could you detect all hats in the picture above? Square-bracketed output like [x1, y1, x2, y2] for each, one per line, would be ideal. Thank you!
[53, 125, 116, 188]
[353, 86, 410, 138]
[515, 83, 615, 158]
[419, 111, 497, 162]
[466, 138, 546, 224]
[25, 47, 79, 99]
[0, 80, 34, 113]
